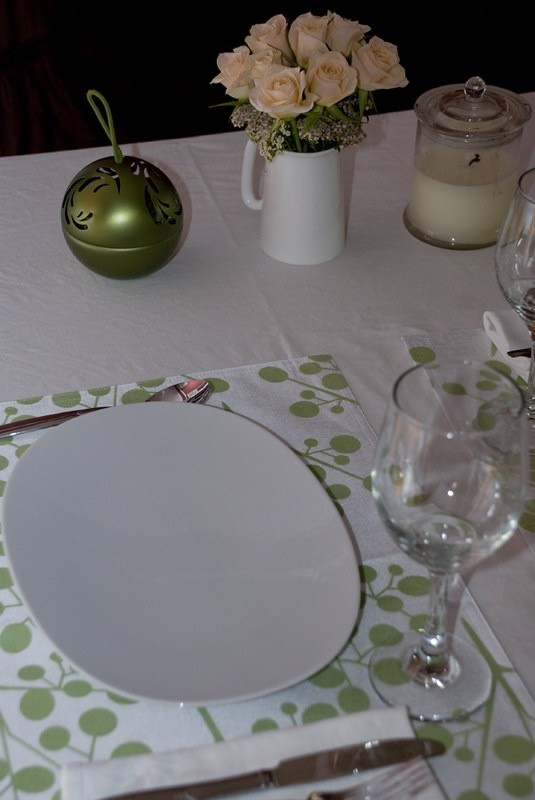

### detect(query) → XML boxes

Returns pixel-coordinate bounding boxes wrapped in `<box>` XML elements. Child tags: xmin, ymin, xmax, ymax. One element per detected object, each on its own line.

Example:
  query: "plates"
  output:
<box><xmin>1</xmin><ymin>402</ymin><xmax>361</xmax><ymax>707</ymax></box>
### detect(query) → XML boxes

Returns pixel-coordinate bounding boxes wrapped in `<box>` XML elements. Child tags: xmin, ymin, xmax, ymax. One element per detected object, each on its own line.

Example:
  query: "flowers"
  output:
<box><xmin>209</xmin><ymin>9</ymin><xmax>411</xmax><ymax>163</ymax></box>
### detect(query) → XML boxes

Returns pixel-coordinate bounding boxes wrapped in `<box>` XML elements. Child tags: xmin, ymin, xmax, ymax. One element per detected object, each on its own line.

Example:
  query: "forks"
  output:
<box><xmin>305</xmin><ymin>754</ymin><xmax>434</xmax><ymax>799</ymax></box>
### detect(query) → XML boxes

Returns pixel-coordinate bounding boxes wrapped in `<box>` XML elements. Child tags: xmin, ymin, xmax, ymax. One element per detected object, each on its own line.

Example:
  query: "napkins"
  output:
<box><xmin>56</xmin><ymin>703</ymin><xmax>443</xmax><ymax>800</ymax></box>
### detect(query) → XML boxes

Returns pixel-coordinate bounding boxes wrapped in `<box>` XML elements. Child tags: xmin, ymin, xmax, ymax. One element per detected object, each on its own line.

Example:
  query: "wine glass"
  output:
<box><xmin>367</xmin><ymin>358</ymin><xmax>529</xmax><ymax>724</ymax></box>
<box><xmin>477</xmin><ymin>166</ymin><xmax>535</xmax><ymax>459</ymax></box>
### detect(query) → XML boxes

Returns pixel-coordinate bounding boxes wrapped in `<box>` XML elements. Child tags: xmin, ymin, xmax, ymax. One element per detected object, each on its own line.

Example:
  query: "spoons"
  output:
<box><xmin>0</xmin><ymin>379</ymin><xmax>215</xmax><ymax>440</ymax></box>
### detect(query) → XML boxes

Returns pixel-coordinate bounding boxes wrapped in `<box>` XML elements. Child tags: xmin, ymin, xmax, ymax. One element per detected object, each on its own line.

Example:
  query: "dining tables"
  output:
<box><xmin>0</xmin><ymin>91</ymin><xmax>535</xmax><ymax>800</ymax></box>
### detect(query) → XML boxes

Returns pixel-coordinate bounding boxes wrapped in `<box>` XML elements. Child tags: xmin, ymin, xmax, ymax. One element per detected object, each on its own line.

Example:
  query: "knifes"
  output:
<box><xmin>103</xmin><ymin>737</ymin><xmax>448</xmax><ymax>800</ymax></box>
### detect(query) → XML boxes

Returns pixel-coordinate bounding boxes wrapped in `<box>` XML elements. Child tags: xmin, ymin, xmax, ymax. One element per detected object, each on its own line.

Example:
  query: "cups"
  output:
<box><xmin>402</xmin><ymin>76</ymin><xmax>532</xmax><ymax>251</ymax></box>
